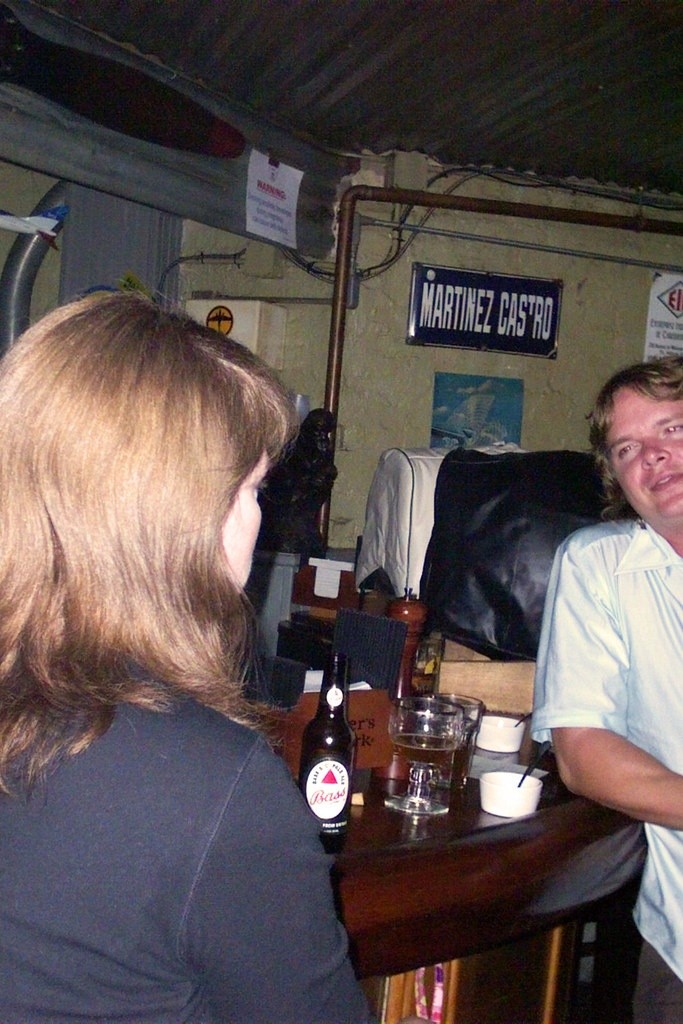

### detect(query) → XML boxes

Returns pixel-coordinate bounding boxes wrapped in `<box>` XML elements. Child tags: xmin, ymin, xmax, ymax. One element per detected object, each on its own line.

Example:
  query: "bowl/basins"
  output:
<box><xmin>477</xmin><ymin>771</ymin><xmax>543</xmax><ymax>818</ymax></box>
<box><xmin>476</xmin><ymin>716</ymin><xmax>526</xmax><ymax>753</ymax></box>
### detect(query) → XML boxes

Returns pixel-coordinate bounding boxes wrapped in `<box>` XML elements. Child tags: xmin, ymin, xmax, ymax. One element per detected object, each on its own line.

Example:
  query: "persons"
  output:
<box><xmin>530</xmin><ymin>353</ymin><xmax>683</xmax><ymax>1024</ymax></box>
<box><xmin>255</xmin><ymin>408</ymin><xmax>338</xmax><ymax>565</ymax></box>
<box><xmin>0</xmin><ymin>288</ymin><xmax>372</xmax><ymax>1024</ymax></box>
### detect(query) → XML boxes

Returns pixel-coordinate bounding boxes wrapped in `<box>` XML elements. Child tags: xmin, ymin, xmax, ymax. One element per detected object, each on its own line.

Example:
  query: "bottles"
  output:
<box><xmin>297</xmin><ymin>651</ymin><xmax>357</xmax><ymax>855</ymax></box>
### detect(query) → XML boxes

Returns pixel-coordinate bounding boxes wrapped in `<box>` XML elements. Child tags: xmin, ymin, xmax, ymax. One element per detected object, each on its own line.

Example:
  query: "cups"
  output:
<box><xmin>410</xmin><ymin>639</ymin><xmax>444</xmax><ymax>696</ymax></box>
<box><xmin>420</xmin><ymin>692</ymin><xmax>483</xmax><ymax>790</ymax></box>
<box><xmin>383</xmin><ymin>697</ymin><xmax>464</xmax><ymax>815</ymax></box>
